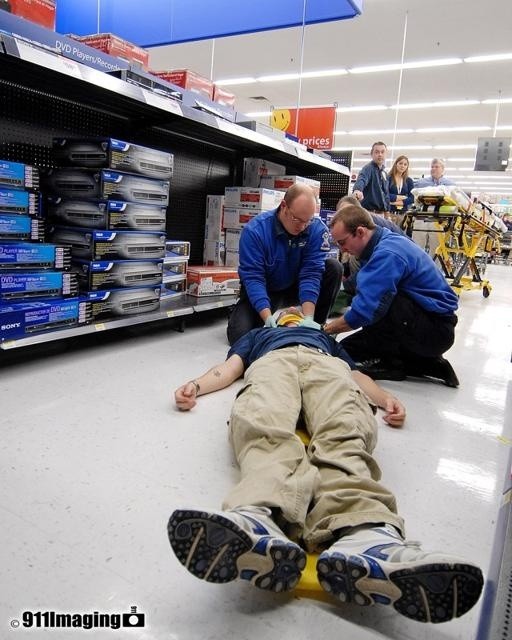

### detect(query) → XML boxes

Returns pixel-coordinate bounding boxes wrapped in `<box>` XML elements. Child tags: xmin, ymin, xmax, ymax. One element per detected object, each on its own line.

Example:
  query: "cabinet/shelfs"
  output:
<box><xmin>0</xmin><ymin>31</ymin><xmax>354</xmax><ymax>352</ymax></box>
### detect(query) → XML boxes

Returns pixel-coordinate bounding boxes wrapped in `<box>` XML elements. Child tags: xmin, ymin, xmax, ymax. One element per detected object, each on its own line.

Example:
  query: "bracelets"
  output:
<box><xmin>188</xmin><ymin>381</ymin><xmax>199</xmax><ymax>398</ymax></box>
<box><xmin>320</xmin><ymin>323</ymin><xmax>327</xmax><ymax>332</ymax></box>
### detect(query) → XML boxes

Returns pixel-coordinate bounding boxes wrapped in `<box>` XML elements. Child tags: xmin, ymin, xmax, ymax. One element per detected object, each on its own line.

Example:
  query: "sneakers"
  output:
<box><xmin>167</xmin><ymin>504</ymin><xmax>306</xmax><ymax>593</ymax></box>
<box><xmin>355</xmin><ymin>359</ymin><xmax>406</xmax><ymax>381</ymax></box>
<box><xmin>316</xmin><ymin>524</ymin><xmax>484</xmax><ymax>624</ymax></box>
<box><xmin>424</xmin><ymin>355</ymin><xmax>459</xmax><ymax>387</ymax></box>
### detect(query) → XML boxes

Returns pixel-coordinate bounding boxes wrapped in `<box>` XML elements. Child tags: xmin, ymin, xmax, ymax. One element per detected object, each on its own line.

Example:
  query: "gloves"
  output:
<box><xmin>265</xmin><ymin>315</ymin><xmax>277</xmax><ymax>328</ymax></box>
<box><xmin>299</xmin><ymin>316</ymin><xmax>321</xmax><ymax>331</ymax></box>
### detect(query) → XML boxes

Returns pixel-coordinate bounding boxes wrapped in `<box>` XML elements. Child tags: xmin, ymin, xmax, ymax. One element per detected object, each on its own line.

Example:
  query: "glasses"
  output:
<box><xmin>336</xmin><ymin>226</ymin><xmax>366</xmax><ymax>246</ymax></box>
<box><xmin>286</xmin><ymin>206</ymin><xmax>313</xmax><ymax>223</ymax></box>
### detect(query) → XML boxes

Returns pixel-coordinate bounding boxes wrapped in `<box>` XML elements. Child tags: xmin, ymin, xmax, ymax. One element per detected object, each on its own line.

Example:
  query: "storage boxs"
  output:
<box><xmin>1</xmin><ymin>9</ymin><xmax>334</xmax><ymax>344</ymax></box>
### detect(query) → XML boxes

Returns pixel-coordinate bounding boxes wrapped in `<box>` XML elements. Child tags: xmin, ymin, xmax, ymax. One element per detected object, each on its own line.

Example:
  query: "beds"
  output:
<box><xmin>399</xmin><ymin>185</ymin><xmax>508</xmax><ymax>298</ymax></box>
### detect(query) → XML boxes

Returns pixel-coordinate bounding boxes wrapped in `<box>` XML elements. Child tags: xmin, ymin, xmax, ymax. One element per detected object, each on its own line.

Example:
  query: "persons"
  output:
<box><xmin>337</xmin><ymin>196</ymin><xmax>415</xmax><ymax>304</ymax></box>
<box><xmin>351</xmin><ymin>141</ymin><xmax>390</xmax><ymax>224</ymax></box>
<box><xmin>384</xmin><ymin>155</ymin><xmax>414</xmax><ymax>238</ymax></box>
<box><xmin>299</xmin><ymin>206</ymin><xmax>459</xmax><ymax>387</ymax></box>
<box><xmin>175</xmin><ymin>306</ymin><xmax>484</xmax><ymax>624</ymax></box>
<box><xmin>411</xmin><ymin>159</ymin><xmax>459</xmax><ymax>278</ymax></box>
<box><xmin>227</xmin><ymin>183</ymin><xmax>344</xmax><ymax>348</ymax></box>
<box><xmin>501</xmin><ymin>213</ymin><xmax>511</xmax><ymax>264</ymax></box>
